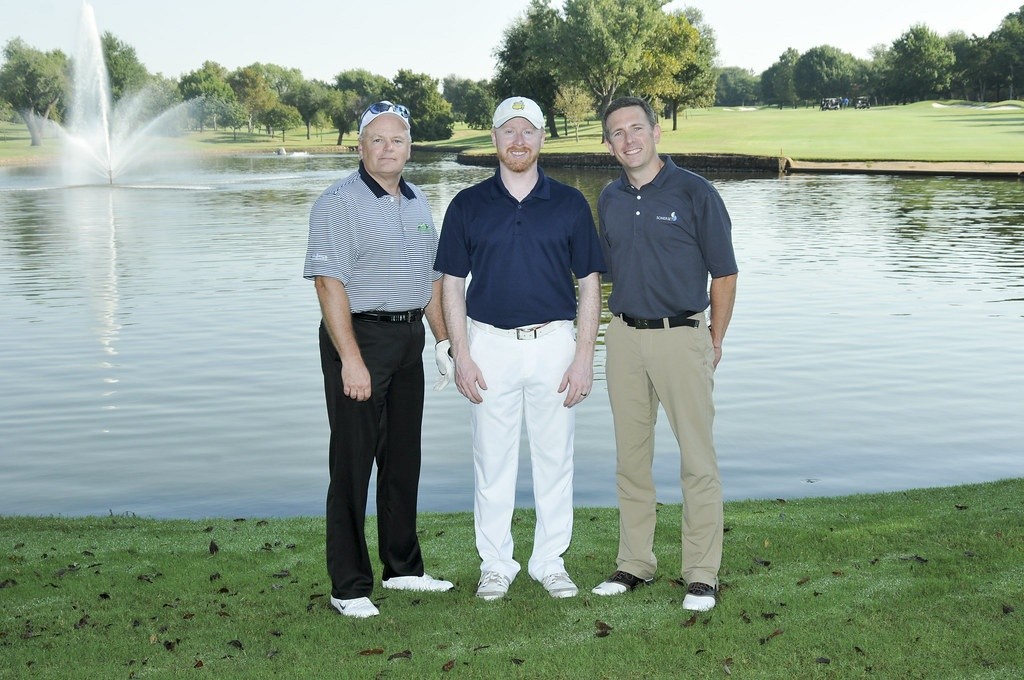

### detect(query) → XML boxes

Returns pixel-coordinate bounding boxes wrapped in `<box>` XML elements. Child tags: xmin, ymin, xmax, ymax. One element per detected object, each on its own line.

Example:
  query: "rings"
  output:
<box><xmin>581</xmin><ymin>393</ymin><xmax>586</xmax><ymax>396</ymax></box>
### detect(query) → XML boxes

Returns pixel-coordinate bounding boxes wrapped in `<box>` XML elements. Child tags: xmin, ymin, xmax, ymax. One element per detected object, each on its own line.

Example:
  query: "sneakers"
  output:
<box><xmin>331</xmin><ymin>594</ymin><xmax>379</xmax><ymax>618</ymax></box>
<box><xmin>540</xmin><ymin>571</ymin><xmax>578</xmax><ymax>597</ymax></box>
<box><xmin>683</xmin><ymin>582</ymin><xmax>716</xmax><ymax>611</ymax></box>
<box><xmin>591</xmin><ymin>569</ymin><xmax>653</xmax><ymax>596</ymax></box>
<box><xmin>382</xmin><ymin>572</ymin><xmax>454</xmax><ymax>593</ymax></box>
<box><xmin>476</xmin><ymin>570</ymin><xmax>511</xmax><ymax>598</ymax></box>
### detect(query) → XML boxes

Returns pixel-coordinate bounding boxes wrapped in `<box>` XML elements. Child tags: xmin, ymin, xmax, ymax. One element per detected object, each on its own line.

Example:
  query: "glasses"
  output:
<box><xmin>358</xmin><ymin>103</ymin><xmax>410</xmax><ymax>127</ymax></box>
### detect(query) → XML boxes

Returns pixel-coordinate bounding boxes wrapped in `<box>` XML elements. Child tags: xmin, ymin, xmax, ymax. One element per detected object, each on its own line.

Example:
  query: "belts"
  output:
<box><xmin>472</xmin><ymin>319</ymin><xmax>568</xmax><ymax>340</ymax></box>
<box><xmin>618</xmin><ymin>310</ymin><xmax>700</xmax><ymax>329</ymax></box>
<box><xmin>351</xmin><ymin>310</ymin><xmax>425</xmax><ymax>323</ymax></box>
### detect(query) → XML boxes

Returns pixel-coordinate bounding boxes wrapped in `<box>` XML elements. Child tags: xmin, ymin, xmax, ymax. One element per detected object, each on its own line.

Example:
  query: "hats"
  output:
<box><xmin>493</xmin><ymin>96</ymin><xmax>545</xmax><ymax>129</ymax></box>
<box><xmin>359</xmin><ymin>100</ymin><xmax>410</xmax><ymax>137</ymax></box>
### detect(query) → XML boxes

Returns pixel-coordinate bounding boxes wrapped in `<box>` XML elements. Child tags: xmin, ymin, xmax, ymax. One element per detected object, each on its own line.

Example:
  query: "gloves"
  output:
<box><xmin>434</xmin><ymin>337</ymin><xmax>455</xmax><ymax>390</ymax></box>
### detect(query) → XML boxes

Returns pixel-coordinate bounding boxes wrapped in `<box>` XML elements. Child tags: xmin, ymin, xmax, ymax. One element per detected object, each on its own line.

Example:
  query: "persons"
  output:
<box><xmin>431</xmin><ymin>97</ymin><xmax>608</xmax><ymax>602</ymax></box>
<box><xmin>590</xmin><ymin>97</ymin><xmax>739</xmax><ymax>612</ymax></box>
<box><xmin>302</xmin><ymin>100</ymin><xmax>457</xmax><ymax>619</ymax></box>
<box><xmin>819</xmin><ymin>97</ymin><xmax>849</xmax><ymax>111</ymax></box>
